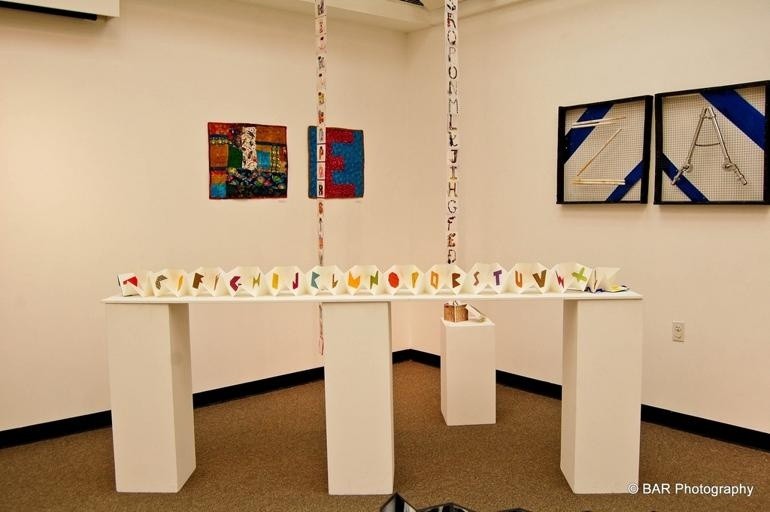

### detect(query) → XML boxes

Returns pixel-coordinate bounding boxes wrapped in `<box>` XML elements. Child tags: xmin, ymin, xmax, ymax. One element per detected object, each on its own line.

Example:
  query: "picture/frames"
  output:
<box><xmin>556</xmin><ymin>80</ymin><xmax>769</xmax><ymax>205</ymax></box>
